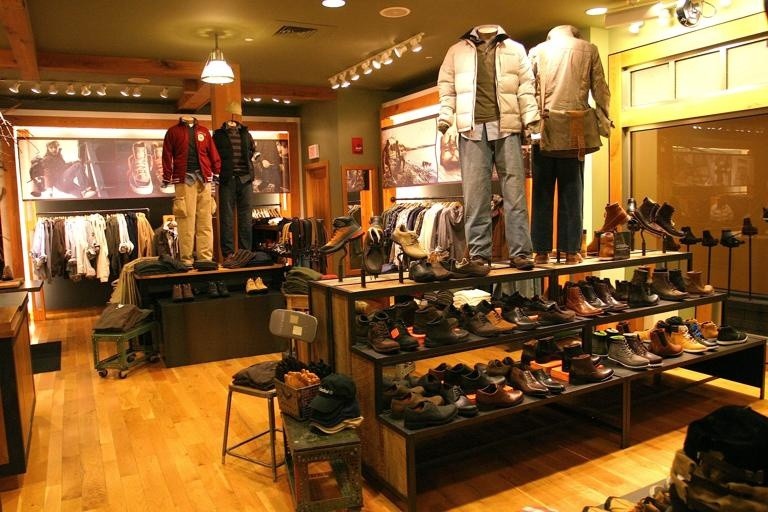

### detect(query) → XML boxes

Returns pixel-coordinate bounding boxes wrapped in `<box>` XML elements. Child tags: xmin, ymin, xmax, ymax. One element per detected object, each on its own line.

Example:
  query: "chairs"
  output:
<box><xmin>221</xmin><ymin>309</ymin><xmax>319</xmax><ymax>482</ymax></box>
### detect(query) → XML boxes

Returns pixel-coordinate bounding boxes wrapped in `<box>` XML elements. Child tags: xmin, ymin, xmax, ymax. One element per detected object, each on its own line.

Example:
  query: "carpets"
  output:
<box><xmin>30</xmin><ymin>341</ymin><xmax>61</xmax><ymax>374</ymax></box>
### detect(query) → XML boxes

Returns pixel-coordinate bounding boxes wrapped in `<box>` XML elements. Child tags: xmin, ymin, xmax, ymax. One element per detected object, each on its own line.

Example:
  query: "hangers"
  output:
<box><xmin>390</xmin><ymin>201</ymin><xmax>463</xmax><ymax>208</ymax></box>
<box><xmin>250</xmin><ymin>207</ymin><xmax>283</xmax><ymax>218</ymax></box>
<box><xmin>37</xmin><ymin>216</ymin><xmax>69</xmax><ymax>220</ymax></box>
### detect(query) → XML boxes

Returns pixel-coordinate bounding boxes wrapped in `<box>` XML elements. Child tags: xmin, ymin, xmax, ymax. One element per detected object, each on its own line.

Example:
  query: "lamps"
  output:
<box><xmin>327</xmin><ymin>32</ymin><xmax>425</xmax><ymax>90</ymax></box>
<box><xmin>8</xmin><ymin>80</ymin><xmax>169</xmax><ymax>99</ymax></box>
<box><xmin>675</xmin><ymin>0</ymin><xmax>705</xmax><ymax>27</ymax></box>
<box><xmin>200</xmin><ymin>33</ymin><xmax>236</xmax><ymax>85</ymax></box>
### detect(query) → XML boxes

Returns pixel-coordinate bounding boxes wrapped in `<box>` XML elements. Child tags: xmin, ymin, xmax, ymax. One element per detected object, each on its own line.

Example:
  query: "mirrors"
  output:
<box><xmin>341</xmin><ymin>165</ymin><xmax>380</xmax><ymax>277</ymax></box>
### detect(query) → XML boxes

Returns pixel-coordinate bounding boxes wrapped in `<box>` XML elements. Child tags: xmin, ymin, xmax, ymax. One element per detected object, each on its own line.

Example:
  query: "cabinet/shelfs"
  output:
<box><xmin>308</xmin><ymin>250</ymin><xmax>767</xmax><ymax>512</ymax></box>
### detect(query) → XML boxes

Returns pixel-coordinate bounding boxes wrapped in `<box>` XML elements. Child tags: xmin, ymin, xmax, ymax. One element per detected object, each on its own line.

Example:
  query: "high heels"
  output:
<box><xmin>588</xmin><ymin>200</ymin><xmax>632</xmax><ymax>232</ymax></box>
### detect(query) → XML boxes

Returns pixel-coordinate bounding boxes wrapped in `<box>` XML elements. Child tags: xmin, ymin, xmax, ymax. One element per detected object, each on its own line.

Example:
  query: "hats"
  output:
<box><xmin>305</xmin><ymin>372</ymin><xmax>365</xmax><ymax>436</ymax></box>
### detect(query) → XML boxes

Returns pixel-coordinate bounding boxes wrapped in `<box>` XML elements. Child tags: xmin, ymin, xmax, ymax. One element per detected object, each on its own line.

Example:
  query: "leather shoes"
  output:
<box><xmin>206</xmin><ymin>279</ymin><xmax>230</xmax><ymax>299</ymax></box>
<box><xmin>171</xmin><ymin>282</ymin><xmax>196</xmax><ymax>303</ymax></box>
<box><xmin>507</xmin><ymin>253</ymin><xmax>550</xmax><ymax>271</ymax></box>
<box><xmin>381</xmin><ymin>355</ymin><xmax>567</xmax><ymax>431</ymax></box>
<box><xmin>563</xmin><ymin>251</ymin><xmax>584</xmax><ymax>266</ymax></box>
<box><xmin>244</xmin><ymin>276</ymin><xmax>268</xmax><ymax>295</ymax></box>
<box><xmin>361</xmin><ymin>224</ymin><xmax>385</xmax><ymax>275</ymax></box>
<box><xmin>390</xmin><ymin>223</ymin><xmax>429</xmax><ymax>261</ymax></box>
<box><xmin>445</xmin><ymin>288</ymin><xmax>577</xmax><ymax>349</ymax></box>
<box><xmin>407</xmin><ymin>253</ymin><xmax>491</xmax><ymax>283</ymax></box>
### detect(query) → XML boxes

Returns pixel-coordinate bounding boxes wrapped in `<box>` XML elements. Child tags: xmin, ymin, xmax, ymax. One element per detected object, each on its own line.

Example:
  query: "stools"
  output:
<box><xmin>283</xmin><ymin>410</ymin><xmax>364</xmax><ymax>512</ymax></box>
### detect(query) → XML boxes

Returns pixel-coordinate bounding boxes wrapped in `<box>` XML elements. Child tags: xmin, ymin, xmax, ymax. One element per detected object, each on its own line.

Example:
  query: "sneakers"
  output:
<box><xmin>315</xmin><ymin>215</ymin><xmax>366</xmax><ymax>254</ymax></box>
<box><xmin>125</xmin><ymin>140</ymin><xmax>176</xmax><ymax>195</ymax></box>
<box><xmin>579</xmin><ymin>475</ymin><xmax>686</xmax><ymax>512</ymax></box>
<box><xmin>585</xmin><ymin>229</ymin><xmax>631</xmax><ymax>261</ymax></box>
<box><xmin>80</xmin><ymin>187</ymin><xmax>97</xmax><ymax>199</ymax></box>
<box><xmin>1</xmin><ymin>265</ymin><xmax>15</xmax><ymax>281</ymax></box>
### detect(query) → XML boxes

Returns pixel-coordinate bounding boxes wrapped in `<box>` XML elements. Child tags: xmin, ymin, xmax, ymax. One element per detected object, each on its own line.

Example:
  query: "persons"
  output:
<box><xmin>213</xmin><ymin>119</ymin><xmax>258</xmax><ymax>264</ymax></box>
<box><xmin>435</xmin><ymin>24</ymin><xmax>536</xmax><ymax>271</ymax></box>
<box><xmin>160</xmin><ymin>114</ymin><xmax>221</xmax><ymax>272</ymax></box>
<box><xmin>527</xmin><ymin>24</ymin><xmax>610</xmax><ymax>267</ymax></box>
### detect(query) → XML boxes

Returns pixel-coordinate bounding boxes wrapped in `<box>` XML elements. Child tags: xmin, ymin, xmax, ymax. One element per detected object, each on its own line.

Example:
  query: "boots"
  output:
<box><xmin>590</xmin><ymin>316</ymin><xmax>750</xmax><ymax>371</ymax></box>
<box><xmin>560</xmin><ymin>341</ymin><xmax>614</xmax><ymax>386</ymax></box>
<box><xmin>632</xmin><ymin>195</ymin><xmax>685</xmax><ymax>239</ymax></box>
<box><xmin>386</xmin><ymin>300</ymin><xmax>470</xmax><ymax>349</ymax></box>
<box><xmin>557</xmin><ymin>265</ymin><xmax>715</xmax><ymax>318</ymax></box>
<box><xmin>354</xmin><ymin>311</ymin><xmax>420</xmax><ymax>353</ymax></box>
<box><xmin>660</xmin><ymin>207</ymin><xmax>767</xmax><ymax>253</ymax></box>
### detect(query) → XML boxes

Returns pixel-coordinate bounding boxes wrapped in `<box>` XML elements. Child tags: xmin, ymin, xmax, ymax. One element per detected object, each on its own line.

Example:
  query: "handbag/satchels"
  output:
<box><xmin>536</xmin><ymin>105</ymin><xmax>603</xmax><ymax>161</ymax></box>
